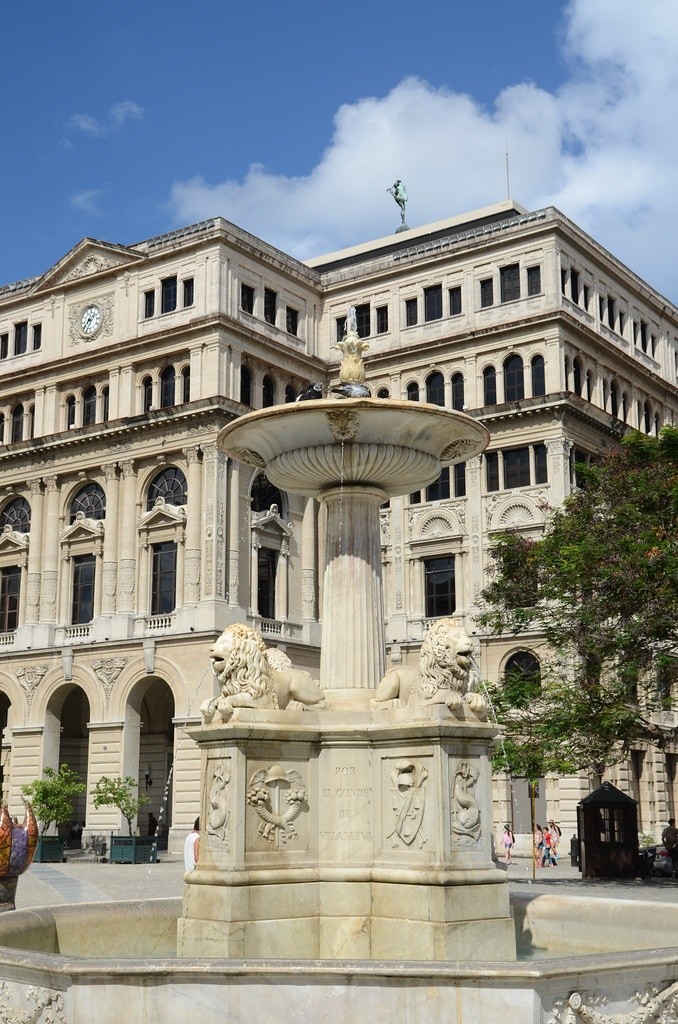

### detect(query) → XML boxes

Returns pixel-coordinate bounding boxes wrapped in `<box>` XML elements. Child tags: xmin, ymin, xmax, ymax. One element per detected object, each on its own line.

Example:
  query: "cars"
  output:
<box><xmin>652</xmin><ymin>846</ymin><xmax>673</xmax><ymax>874</ymax></box>
<box><xmin>639</xmin><ymin>843</ymin><xmax>664</xmax><ymax>868</ymax></box>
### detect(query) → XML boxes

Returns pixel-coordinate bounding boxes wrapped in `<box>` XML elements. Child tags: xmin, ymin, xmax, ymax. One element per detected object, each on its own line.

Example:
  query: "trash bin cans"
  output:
<box><xmin>97</xmin><ymin>842</ymin><xmax>106</xmax><ymax>856</ymax></box>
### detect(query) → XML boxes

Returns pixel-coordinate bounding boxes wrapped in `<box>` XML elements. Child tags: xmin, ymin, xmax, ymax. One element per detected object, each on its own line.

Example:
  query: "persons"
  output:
<box><xmin>147</xmin><ymin>812</ymin><xmax>157</xmax><ymax>837</ymax></box>
<box><xmin>63</xmin><ymin>821</ymin><xmax>85</xmax><ymax>847</ymax></box>
<box><xmin>500</xmin><ymin>824</ymin><xmax>514</xmax><ymax>865</ymax></box>
<box><xmin>184</xmin><ymin>816</ymin><xmax>200</xmax><ymax>872</ymax></box>
<box><xmin>662</xmin><ymin>819</ymin><xmax>678</xmax><ymax>879</ymax></box>
<box><xmin>534</xmin><ymin>819</ymin><xmax>560</xmax><ymax>867</ymax></box>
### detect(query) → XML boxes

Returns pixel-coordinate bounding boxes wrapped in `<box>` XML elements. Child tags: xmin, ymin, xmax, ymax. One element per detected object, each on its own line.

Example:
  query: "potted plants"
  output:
<box><xmin>93</xmin><ymin>775</ymin><xmax>154</xmax><ymax>865</ymax></box>
<box><xmin>23</xmin><ymin>764</ymin><xmax>85</xmax><ymax>862</ymax></box>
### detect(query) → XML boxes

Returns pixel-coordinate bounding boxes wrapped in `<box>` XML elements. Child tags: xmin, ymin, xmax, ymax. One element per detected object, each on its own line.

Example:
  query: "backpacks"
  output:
<box><xmin>554</xmin><ymin>825</ymin><xmax>561</xmax><ymax>836</ymax></box>
<box><xmin>508</xmin><ymin>830</ymin><xmax>515</xmax><ymax>843</ymax></box>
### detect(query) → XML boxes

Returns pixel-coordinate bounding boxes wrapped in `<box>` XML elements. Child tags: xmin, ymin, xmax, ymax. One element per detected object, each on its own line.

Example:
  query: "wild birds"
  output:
<box><xmin>295</xmin><ymin>383</ymin><xmax>324</xmax><ymax>402</ymax></box>
<box><xmin>331</xmin><ymin>384</ymin><xmax>372</xmax><ymax>398</ymax></box>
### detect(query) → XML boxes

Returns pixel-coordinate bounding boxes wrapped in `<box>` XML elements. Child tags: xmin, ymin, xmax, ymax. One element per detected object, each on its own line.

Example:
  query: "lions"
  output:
<box><xmin>373</xmin><ymin>617</ymin><xmax>488</xmax><ymax>713</ymax></box>
<box><xmin>199</xmin><ymin>623</ymin><xmax>327</xmax><ymax>725</ymax></box>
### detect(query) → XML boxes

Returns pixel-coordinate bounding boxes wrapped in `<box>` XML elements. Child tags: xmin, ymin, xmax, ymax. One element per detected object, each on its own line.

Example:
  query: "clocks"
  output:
<box><xmin>78</xmin><ymin>302</ymin><xmax>102</xmax><ymax>338</ymax></box>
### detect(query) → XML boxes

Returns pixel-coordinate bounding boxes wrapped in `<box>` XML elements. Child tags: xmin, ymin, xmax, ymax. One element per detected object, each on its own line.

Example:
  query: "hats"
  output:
<box><xmin>504</xmin><ymin>824</ymin><xmax>510</xmax><ymax>829</ymax></box>
<box><xmin>547</xmin><ymin>819</ymin><xmax>554</xmax><ymax>823</ymax></box>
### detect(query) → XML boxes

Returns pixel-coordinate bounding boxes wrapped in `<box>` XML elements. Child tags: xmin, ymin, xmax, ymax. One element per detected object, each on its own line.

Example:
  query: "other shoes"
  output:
<box><xmin>548</xmin><ymin>864</ymin><xmax>553</xmax><ymax>866</ymax></box>
<box><xmin>540</xmin><ymin>864</ymin><xmax>544</xmax><ymax>866</ymax></box>
